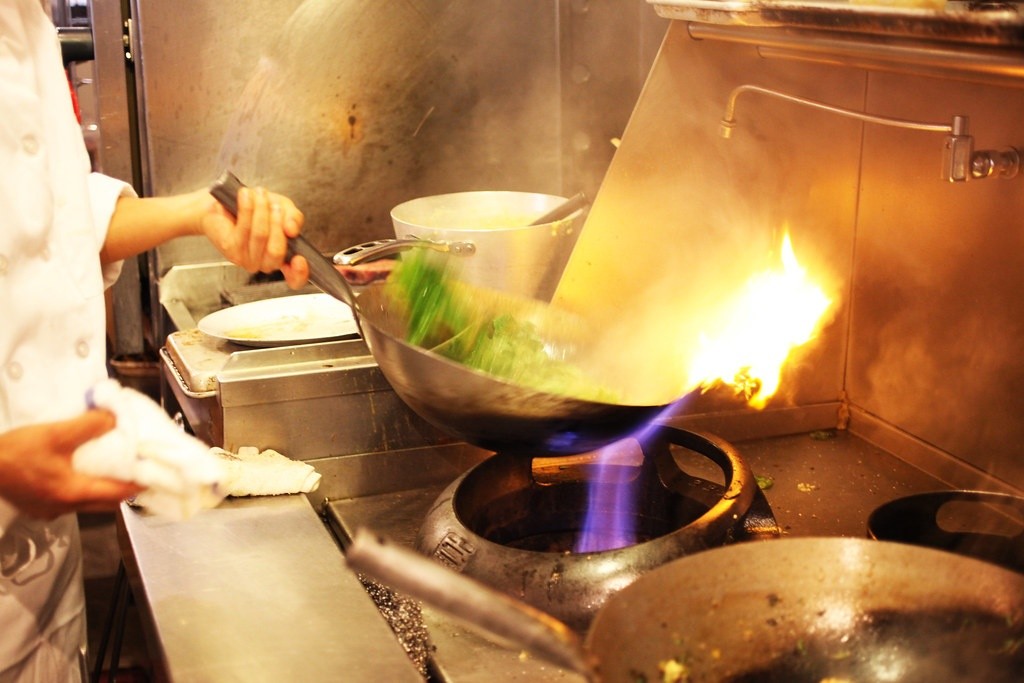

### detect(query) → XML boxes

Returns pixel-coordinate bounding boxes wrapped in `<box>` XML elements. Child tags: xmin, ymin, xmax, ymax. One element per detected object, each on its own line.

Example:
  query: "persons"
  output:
<box><xmin>0</xmin><ymin>1</ymin><xmax>309</xmax><ymax>683</ymax></box>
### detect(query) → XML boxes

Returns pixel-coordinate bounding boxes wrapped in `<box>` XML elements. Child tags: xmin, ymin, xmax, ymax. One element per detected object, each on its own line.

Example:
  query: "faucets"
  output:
<box><xmin>718</xmin><ymin>84</ymin><xmax>967</xmax><ymax>139</ymax></box>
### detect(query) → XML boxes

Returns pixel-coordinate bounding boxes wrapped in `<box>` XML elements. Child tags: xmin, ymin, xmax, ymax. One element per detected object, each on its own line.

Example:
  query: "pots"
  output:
<box><xmin>346</xmin><ymin>524</ymin><xmax>1024</xmax><ymax>683</ymax></box>
<box><xmin>210</xmin><ymin>171</ymin><xmax>687</xmax><ymax>457</ymax></box>
<box><xmin>334</xmin><ymin>192</ymin><xmax>582</xmax><ymax>302</ymax></box>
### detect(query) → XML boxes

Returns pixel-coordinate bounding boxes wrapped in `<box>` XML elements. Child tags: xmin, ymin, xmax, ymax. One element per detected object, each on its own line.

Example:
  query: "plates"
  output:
<box><xmin>199</xmin><ymin>293</ymin><xmax>358</xmax><ymax>348</ymax></box>
